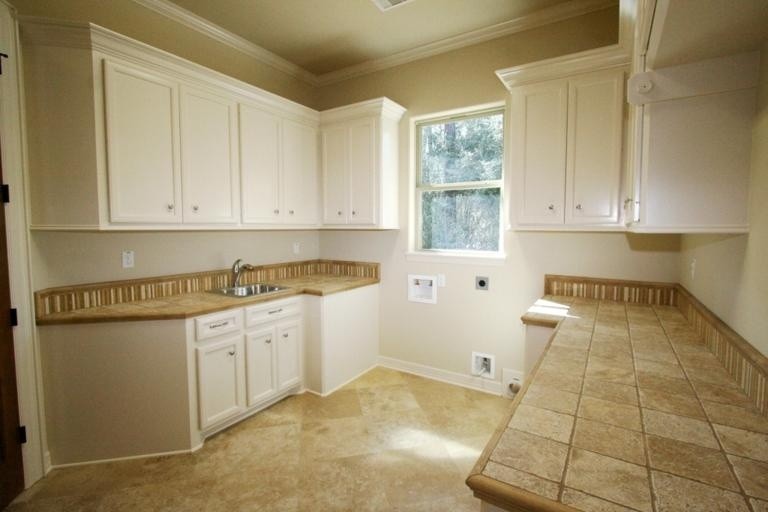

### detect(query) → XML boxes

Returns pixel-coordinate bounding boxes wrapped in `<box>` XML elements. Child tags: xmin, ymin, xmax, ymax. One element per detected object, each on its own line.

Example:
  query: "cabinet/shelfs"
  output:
<box><xmin>237</xmin><ymin>98</ymin><xmax>319</xmax><ymax>230</ymax></box>
<box><xmin>38</xmin><ymin>307</ymin><xmax>246</xmax><ymax>468</ymax></box>
<box><xmin>321</xmin><ymin>116</ymin><xmax>402</xmax><ymax>231</ymax></box>
<box><xmin>24</xmin><ymin>37</ymin><xmax>236</xmax><ymax>230</ymax></box>
<box><xmin>245</xmin><ymin>294</ymin><xmax>310</xmax><ymax>409</ymax></box>
<box><xmin>506</xmin><ymin>0</ymin><xmax>749</xmax><ymax>231</ymax></box>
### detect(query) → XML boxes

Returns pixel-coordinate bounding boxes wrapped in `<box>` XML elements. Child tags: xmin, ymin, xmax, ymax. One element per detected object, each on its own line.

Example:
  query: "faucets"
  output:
<box><xmin>232</xmin><ymin>258</ymin><xmax>253</xmax><ymax>288</ymax></box>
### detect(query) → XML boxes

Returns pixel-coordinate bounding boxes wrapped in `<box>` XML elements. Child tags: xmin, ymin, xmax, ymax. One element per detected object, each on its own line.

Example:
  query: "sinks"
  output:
<box><xmin>204</xmin><ymin>284</ymin><xmax>291</xmax><ymax>297</ymax></box>
